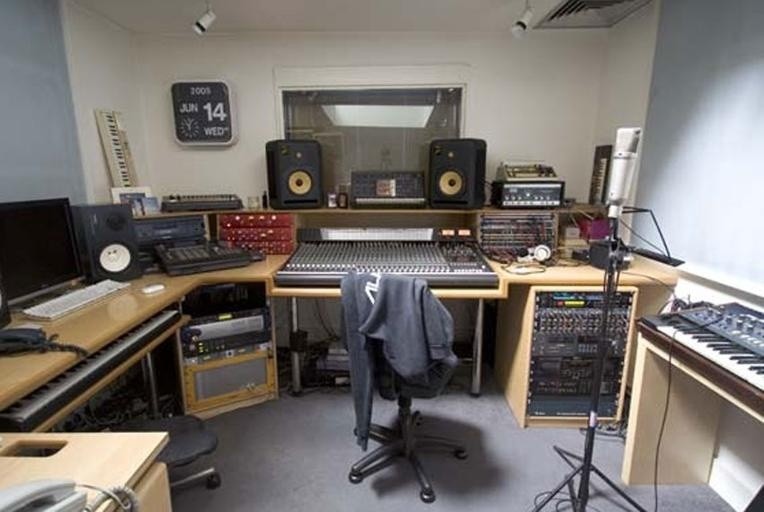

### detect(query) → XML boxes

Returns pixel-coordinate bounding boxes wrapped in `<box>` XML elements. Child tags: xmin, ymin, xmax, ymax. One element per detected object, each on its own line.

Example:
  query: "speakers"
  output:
<box><xmin>428</xmin><ymin>138</ymin><xmax>487</xmax><ymax>210</ymax></box>
<box><xmin>266</xmin><ymin>140</ymin><xmax>323</xmax><ymax>209</ymax></box>
<box><xmin>71</xmin><ymin>203</ymin><xmax>142</xmax><ymax>285</ymax></box>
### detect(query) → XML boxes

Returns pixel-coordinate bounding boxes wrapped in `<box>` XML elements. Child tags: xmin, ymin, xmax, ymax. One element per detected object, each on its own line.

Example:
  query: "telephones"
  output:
<box><xmin>0</xmin><ymin>474</ymin><xmax>88</xmax><ymax>511</ymax></box>
<box><xmin>0</xmin><ymin>328</ymin><xmax>46</xmax><ymax>355</ymax></box>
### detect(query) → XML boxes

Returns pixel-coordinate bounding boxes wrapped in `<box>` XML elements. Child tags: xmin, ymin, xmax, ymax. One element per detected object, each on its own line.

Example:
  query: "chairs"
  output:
<box><xmin>336</xmin><ymin>274</ymin><xmax>472</xmax><ymax>504</ymax></box>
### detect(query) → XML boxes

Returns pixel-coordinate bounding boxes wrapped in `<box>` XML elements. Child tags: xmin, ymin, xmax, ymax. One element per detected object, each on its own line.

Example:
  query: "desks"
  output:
<box><xmin>1</xmin><ymin>253</ymin><xmax>677</xmax><ymax>434</ymax></box>
<box><xmin>622</xmin><ymin>327</ymin><xmax>764</xmax><ymax>489</ymax></box>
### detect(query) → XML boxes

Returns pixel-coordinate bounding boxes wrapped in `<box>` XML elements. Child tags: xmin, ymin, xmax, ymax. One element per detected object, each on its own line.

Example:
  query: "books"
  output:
<box><xmin>479</xmin><ymin>211</ymin><xmax>555</xmax><ymax>254</ymax></box>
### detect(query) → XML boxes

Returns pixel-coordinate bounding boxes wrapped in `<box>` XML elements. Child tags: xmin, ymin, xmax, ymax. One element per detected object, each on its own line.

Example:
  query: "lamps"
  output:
<box><xmin>512</xmin><ymin>0</ymin><xmax>535</xmax><ymax>33</ymax></box>
<box><xmin>192</xmin><ymin>2</ymin><xmax>216</xmax><ymax>34</ymax></box>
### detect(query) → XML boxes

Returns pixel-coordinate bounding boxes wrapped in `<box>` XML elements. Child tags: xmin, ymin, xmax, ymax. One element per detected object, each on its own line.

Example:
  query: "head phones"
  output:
<box><xmin>517</xmin><ymin>244</ymin><xmax>551</xmax><ymax>263</ymax></box>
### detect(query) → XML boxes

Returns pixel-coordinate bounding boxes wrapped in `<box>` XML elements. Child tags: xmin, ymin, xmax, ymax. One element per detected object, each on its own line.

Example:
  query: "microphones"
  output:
<box><xmin>607</xmin><ymin>127</ymin><xmax>644</xmax><ymax>219</ymax></box>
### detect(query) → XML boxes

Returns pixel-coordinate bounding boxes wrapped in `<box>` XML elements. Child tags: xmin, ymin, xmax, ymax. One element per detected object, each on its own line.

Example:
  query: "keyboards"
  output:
<box><xmin>21</xmin><ymin>278</ymin><xmax>132</xmax><ymax>320</ymax></box>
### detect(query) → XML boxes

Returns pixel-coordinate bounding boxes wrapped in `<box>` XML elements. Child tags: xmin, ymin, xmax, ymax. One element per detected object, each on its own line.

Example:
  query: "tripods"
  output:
<box><xmin>532</xmin><ymin>218</ymin><xmax>685</xmax><ymax>512</ymax></box>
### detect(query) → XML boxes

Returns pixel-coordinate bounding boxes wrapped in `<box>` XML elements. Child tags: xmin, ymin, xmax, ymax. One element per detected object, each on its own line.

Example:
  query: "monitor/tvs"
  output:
<box><xmin>0</xmin><ymin>197</ymin><xmax>86</xmax><ymax>306</ymax></box>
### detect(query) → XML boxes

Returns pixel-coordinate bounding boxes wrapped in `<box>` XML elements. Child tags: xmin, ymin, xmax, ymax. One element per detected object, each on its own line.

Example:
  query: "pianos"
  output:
<box><xmin>640</xmin><ymin>302</ymin><xmax>764</xmax><ymax>398</ymax></box>
<box><xmin>0</xmin><ymin>308</ymin><xmax>181</xmax><ymax>433</ymax></box>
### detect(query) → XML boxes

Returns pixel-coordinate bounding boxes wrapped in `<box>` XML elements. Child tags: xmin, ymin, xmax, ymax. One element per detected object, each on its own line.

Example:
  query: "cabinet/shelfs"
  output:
<box><xmin>0</xmin><ymin>433</ymin><xmax>175</xmax><ymax>512</ymax></box>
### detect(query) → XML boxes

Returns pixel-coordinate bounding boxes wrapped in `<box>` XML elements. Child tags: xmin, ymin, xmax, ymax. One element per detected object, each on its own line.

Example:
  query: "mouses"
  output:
<box><xmin>142</xmin><ymin>284</ymin><xmax>165</xmax><ymax>294</ymax></box>
